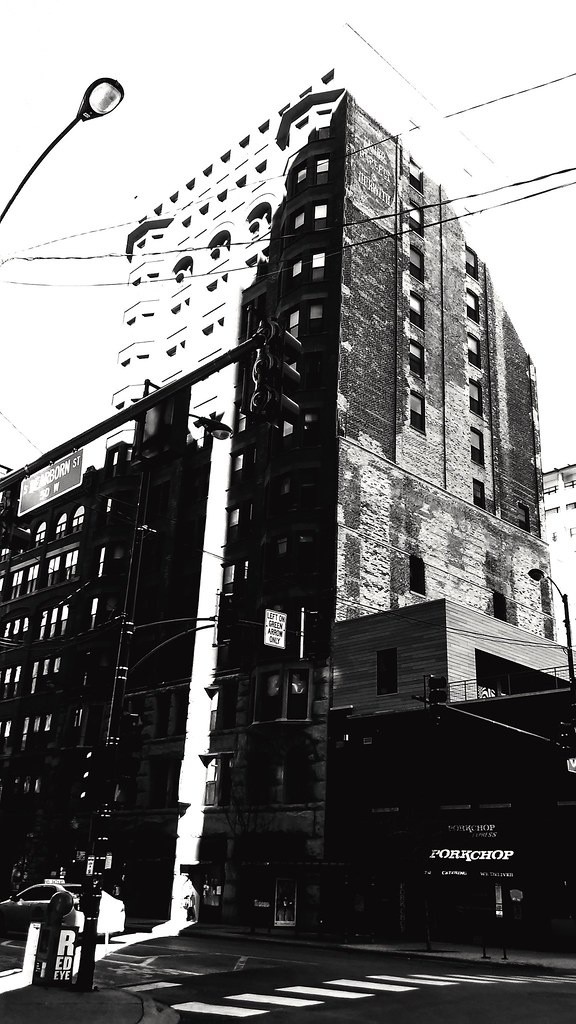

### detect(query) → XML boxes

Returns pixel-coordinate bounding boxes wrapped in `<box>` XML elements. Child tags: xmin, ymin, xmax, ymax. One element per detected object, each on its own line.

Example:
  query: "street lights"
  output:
<box><xmin>527</xmin><ymin>568</ymin><xmax>576</xmax><ymax>720</ymax></box>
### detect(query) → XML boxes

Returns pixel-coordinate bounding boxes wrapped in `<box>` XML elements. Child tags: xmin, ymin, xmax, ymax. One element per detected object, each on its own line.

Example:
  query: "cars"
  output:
<box><xmin>0</xmin><ymin>879</ymin><xmax>126</xmax><ymax>944</ymax></box>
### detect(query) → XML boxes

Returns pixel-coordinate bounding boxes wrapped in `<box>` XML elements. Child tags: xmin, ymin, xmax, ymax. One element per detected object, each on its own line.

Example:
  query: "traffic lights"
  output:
<box><xmin>427</xmin><ymin>673</ymin><xmax>448</xmax><ymax>728</ymax></box>
<box><xmin>248</xmin><ymin>318</ymin><xmax>302</xmax><ymax>437</ymax></box>
<box><xmin>303</xmin><ymin>608</ymin><xmax>320</xmax><ymax>659</ymax></box>
<box><xmin>217</xmin><ymin>591</ymin><xmax>237</xmax><ymax>645</ymax></box>
